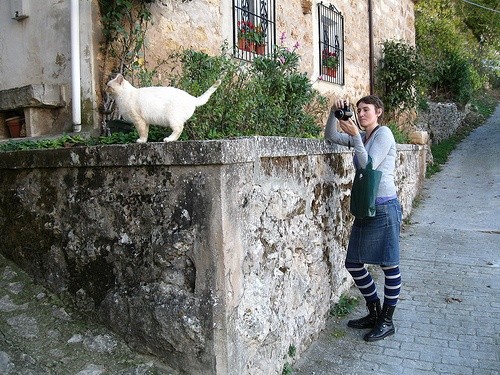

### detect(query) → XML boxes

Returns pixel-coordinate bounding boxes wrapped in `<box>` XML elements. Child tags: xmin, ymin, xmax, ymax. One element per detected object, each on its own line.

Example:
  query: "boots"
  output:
<box><xmin>363</xmin><ymin>302</ymin><xmax>398</xmax><ymax>344</ymax></box>
<box><xmin>347</xmin><ymin>299</ymin><xmax>381</xmax><ymax>329</ymax></box>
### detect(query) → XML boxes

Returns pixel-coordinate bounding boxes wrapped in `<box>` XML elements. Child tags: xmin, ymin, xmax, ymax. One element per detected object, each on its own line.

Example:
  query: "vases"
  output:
<box><xmin>256</xmin><ymin>44</ymin><xmax>266</xmax><ymax>56</ymax></box>
<box><xmin>238</xmin><ymin>38</ymin><xmax>248</xmax><ymax>50</ymax></box>
<box><xmin>246</xmin><ymin>42</ymin><xmax>256</xmax><ymax>52</ymax></box>
<box><xmin>323</xmin><ymin>66</ymin><xmax>336</xmax><ymax>77</ymax></box>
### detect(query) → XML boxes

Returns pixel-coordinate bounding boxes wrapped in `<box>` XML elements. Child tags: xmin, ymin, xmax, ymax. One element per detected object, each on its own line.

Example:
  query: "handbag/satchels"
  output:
<box><xmin>350</xmin><ymin>154</ymin><xmax>382</xmax><ymax>220</ymax></box>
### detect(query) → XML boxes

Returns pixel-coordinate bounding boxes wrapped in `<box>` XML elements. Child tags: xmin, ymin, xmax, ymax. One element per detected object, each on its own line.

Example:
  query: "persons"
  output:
<box><xmin>324</xmin><ymin>96</ymin><xmax>401</xmax><ymax>342</ymax></box>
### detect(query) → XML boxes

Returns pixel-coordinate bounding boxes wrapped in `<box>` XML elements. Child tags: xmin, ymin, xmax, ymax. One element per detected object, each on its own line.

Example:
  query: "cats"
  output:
<box><xmin>108</xmin><ymin>68</ymin><xmax>228</xmax><ymax>143</ymax></box>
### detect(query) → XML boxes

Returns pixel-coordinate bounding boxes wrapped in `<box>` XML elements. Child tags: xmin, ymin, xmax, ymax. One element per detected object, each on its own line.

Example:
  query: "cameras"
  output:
<box><xmin>334</xmin><ymin>103</ymin><xmax>352</xmax><ymax>121</ymax></box>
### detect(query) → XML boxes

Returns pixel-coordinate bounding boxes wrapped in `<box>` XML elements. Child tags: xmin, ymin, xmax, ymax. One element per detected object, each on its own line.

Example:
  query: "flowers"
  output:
<box><xmin>322</xmin><ymin>48</ymin><xmax>338</xmax><ymax>68</ymax></box>
<box><xmin>238</xmin><ymin>20</ymin><xmax>267</xmax><ymax>45</ymax></box>
